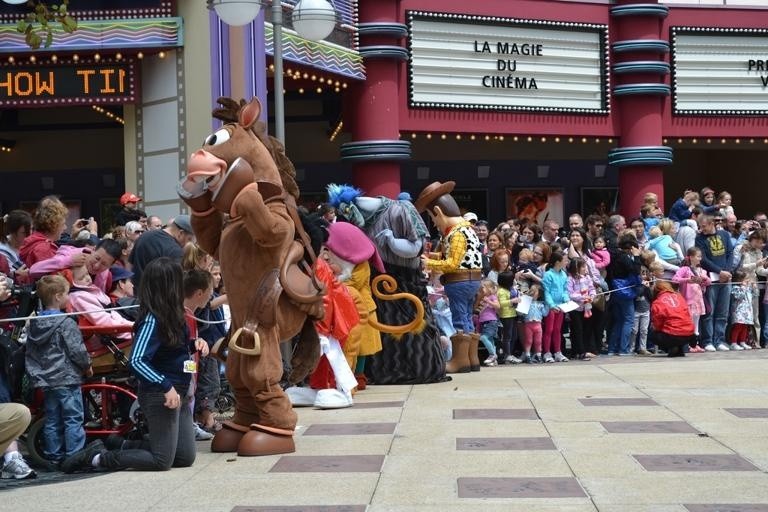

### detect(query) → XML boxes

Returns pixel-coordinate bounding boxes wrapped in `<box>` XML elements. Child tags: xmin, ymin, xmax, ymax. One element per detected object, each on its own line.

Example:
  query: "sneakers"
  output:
<box><xmin>104</xmin><ymin>435</ymin><xmax>124</xmax><ymax>450</ymax></box>
<box><xmin>486</xmin><ymin>359</ymin><xmax>499</xmax><ymax>367</ymax></box>
<box><xmin>505</xmin><ymin>354</ymin><xmax>524</xmax><ymax>365</ymax></box>
<box><xmin>483</xmin><ymin>353</ymin><xmax>498</xmax><ymax>364</ymax></box>
<box><xmin>571</xmin><ymin>349</ymin><xmax>654</xmax><ymax>361</ymax></box>
<box><xmin>688</xmin><ymin>341</ymin><xmax>763</xmax><ymax>353</ymax></box>
<box><xmin>543</xmin><ymin>352</ymin><xmax>556</xmax><ymax>363</ymax></box>
<box><xmin>1</xmin><ymin>453</ymin><xmax>39</xmax><ymax>480</ymax></box>
<box><xmin>60</xmin><ymin>438</ymin><xmax>105</xmax><ymax>472</ymax></box>
<box><xmin>520</xmin><ymin>352</ymin><xmax>544</xmax><ymax>364</ymax></box>
<box><xmin>554</xmin><ymin>351</ymin><xmax>570</xmax><ymax>362</ymax></box>
<box><xmin>193</xmin><ymin>423</ymin><xmax>214</xmax><ymax>441</ymax></box>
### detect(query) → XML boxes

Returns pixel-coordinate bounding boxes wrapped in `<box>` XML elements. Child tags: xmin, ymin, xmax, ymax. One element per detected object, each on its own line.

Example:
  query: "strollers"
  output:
<box><xmin>9</xmin><ymin>308</ymin><xmax>240</xmax><ymax>470</ymax></box>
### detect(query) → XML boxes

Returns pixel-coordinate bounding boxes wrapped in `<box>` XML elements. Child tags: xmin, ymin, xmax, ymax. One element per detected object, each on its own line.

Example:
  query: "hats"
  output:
<box><xmin>174</xmin><ymin>215</ymin><xmax>193</xmax><ymax>234</ymax></box>
<box><xmin>119</xmin><ymin>191</ymin><xmax>144</xmax><ymax>206</ymax></box>
<box><xmin>109</xmin><ymin>265</ymin><xmax>136</xmax><ymax>282</ymax></box>
<box><xmin>463</xmin><ymin>212</ymin><xmax>479</xmax><ymax>223</ymax></box>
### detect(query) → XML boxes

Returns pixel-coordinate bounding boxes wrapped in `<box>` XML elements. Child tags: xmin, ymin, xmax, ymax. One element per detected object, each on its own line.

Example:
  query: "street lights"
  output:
<box><xmin>205</xmin><ymin>0</ymin><xmax>337</xmax><ymax>157</ymax></box>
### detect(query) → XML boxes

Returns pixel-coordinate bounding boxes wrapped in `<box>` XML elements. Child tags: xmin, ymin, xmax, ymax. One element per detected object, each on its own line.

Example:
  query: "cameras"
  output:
<box><xmin>520</xmin><ymin>235</ymin><xmax>527</xmax><ymax>241</ymax></box>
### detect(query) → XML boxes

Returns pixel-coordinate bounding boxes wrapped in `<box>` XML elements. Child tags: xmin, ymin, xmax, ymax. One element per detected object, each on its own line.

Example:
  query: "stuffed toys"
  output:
<box><xmin>175</xmin><ymin>96</ymin><xmax>325</xmax><ymax>458</ymax></box>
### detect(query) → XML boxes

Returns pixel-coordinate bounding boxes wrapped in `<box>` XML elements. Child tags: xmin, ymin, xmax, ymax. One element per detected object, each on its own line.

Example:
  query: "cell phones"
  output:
<box><xmin>12</xmin><ymin>261</ymin><xmax>23</xmax><ymax>269</ymax></box>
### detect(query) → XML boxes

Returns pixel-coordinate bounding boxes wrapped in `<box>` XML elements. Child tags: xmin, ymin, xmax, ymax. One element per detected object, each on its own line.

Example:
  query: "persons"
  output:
<box><xmin>424</xmin><ymin>187</ymin><xmax>767</xmax><ymax>385</ymax></box>
<box><xmin>0</xmin><ymin>191</ymin><xmax>233</xmax><ymax>481</ymax></box>
